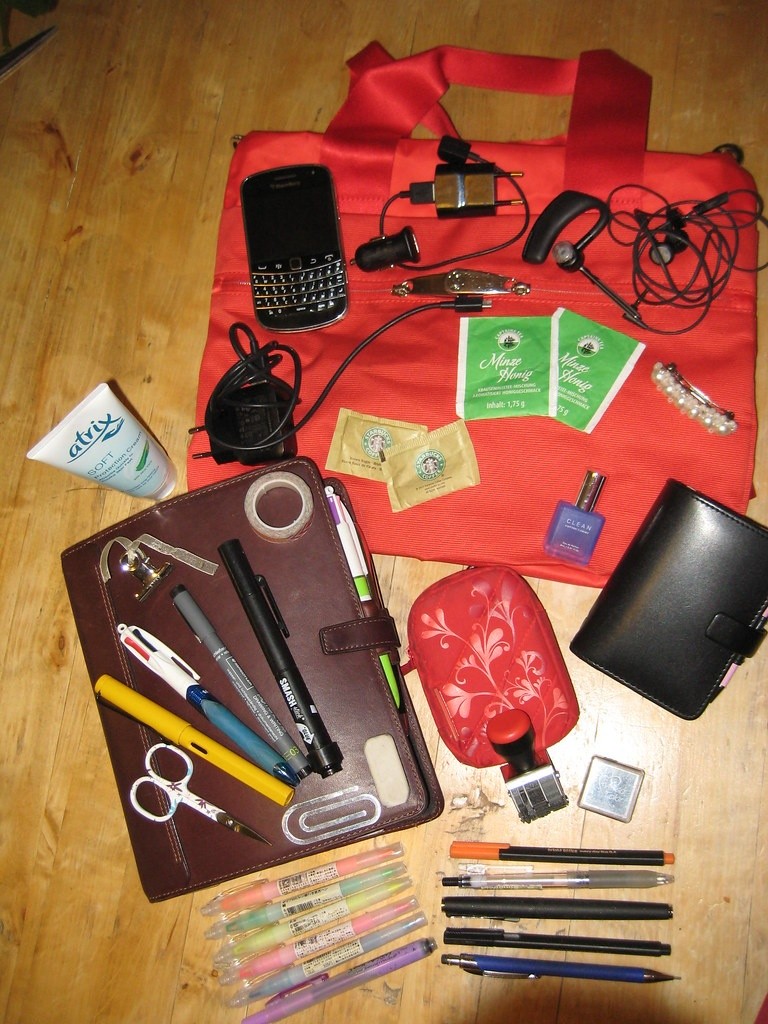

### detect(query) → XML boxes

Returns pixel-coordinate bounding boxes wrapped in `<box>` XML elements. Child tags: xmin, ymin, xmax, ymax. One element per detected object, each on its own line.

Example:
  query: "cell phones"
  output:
<box><xmin>240</xmin><ymin>165</ymin><xmax>350</xmax><ymax>334</ymax></box>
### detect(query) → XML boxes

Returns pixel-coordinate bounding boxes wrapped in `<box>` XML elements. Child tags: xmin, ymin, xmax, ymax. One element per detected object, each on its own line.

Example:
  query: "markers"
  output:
<box><xmin>240</xmin><ymin>936</ymin><xmax>438</xmax><ymax>1024</ymax></box>
<box><xmin>199</xmin><ymin>839</ymin><xmax>428</xmax><ymax>1007</ymax></box>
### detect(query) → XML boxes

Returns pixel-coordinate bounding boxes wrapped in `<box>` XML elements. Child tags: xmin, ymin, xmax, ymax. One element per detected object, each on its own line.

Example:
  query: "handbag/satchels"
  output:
<box><xmin>186</xmin><ymin>40</ymin><xmax>757</xmax><ymax>584</ymax></box>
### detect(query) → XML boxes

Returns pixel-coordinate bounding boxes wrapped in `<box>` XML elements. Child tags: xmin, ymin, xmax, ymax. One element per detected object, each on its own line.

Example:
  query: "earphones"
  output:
<box><xmin>663</xmin><ymin>210</ymin><xmax>690</xmax><ymax>255</ymax></box>
<box><xmin>650</xmin><ymin>236</ymin><xmax>672</xmax><ymax>265</ymax></box>
<box><xmin>552</xmin><ymin>241</ymin><xmax>586</xmax><ymax>272</ymax></box>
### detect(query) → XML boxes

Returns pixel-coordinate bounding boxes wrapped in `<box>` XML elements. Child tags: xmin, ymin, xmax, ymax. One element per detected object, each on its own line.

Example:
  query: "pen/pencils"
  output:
<box><xmin>443</xmin><ymin>926</ymin><xmax>672</xmax><ymax>957</ymax></box>
<box><xmin>450</xmin><ymin>839</ymin><xmax>675</xmax><ymax>867</ymax></box>
<box><xmin>440</xmin><ymin>953</ymin><xmax>682</xmax><ymax>984</ymax></box>
<box><xmin>440</xmin><ymin>896</ymin><xmax>673</xmax><ymax>922</ymax></box>
<box><xmin>442</xmin><ymin>870</ymin><xmax>675</xmax><ymax>889</ymax></box>
<box><xmin>116</xmin><ymin>622</ymin><xmax>300</xmax><ymax>789</ymax></box>
<box><xmin>216</xmin><ymin>539</ymin><xmax>345</xmax><ymax>781</ymax></box>
<box><xmin>92</xmin><ymin>673</ymin><xmax>296</xmax><ymax>809</ymax></box>
<box><xmin>324</xmin><ymin>485</ymin><xmax>400</xmax><ymax>709</ymax></box>
<box><xmin>168</xmin><ymin>583</ymin><xmax>313</xmax><ymax>782</ymax></box>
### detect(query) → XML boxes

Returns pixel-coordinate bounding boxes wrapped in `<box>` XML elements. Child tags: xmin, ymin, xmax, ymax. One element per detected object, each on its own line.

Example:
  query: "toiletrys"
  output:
<box><xmin>26</xmin><ymin>383</ymin><xmax>177</xmax><ymax>501</ymax></box>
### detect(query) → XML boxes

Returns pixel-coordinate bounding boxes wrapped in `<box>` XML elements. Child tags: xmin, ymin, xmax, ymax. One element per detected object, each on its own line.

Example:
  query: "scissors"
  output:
<box><xmin>128</xmin><ymin>742</ymin><xmax>273</xmax><ymax>848</ymax></box>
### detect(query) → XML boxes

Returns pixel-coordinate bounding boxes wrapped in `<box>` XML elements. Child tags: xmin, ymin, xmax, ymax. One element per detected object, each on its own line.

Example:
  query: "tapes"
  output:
<box><xmin>243</xmin><ymin>471</ymin><xmax>314</xmax><ymax>545</ymax></box>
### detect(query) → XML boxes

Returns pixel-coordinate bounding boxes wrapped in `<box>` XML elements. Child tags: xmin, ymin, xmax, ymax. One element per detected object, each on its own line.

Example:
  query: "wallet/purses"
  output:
<box><xmin>406</xmin><ymin>566</ymin><xmax>580</xmax><ymax>769</ymax></box>
